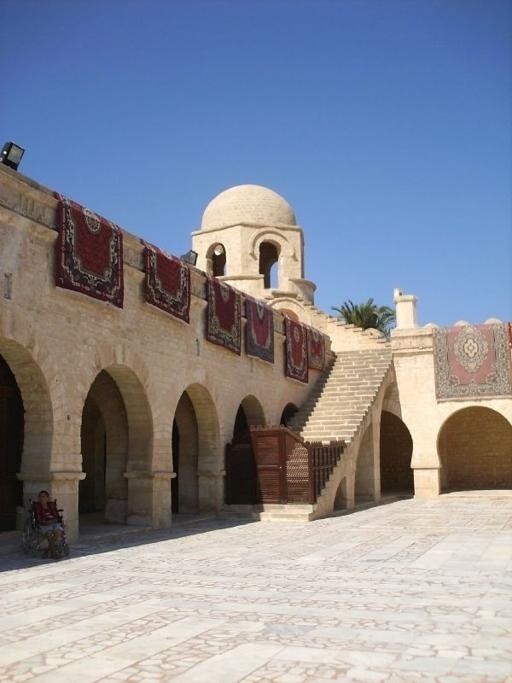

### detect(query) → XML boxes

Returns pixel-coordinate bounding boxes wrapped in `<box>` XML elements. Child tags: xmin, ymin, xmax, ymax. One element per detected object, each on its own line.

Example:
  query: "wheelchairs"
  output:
<box><xmin>22</xmin><ymin>495</ymin><xmax>70</xmax><ymax>559</ymax></box>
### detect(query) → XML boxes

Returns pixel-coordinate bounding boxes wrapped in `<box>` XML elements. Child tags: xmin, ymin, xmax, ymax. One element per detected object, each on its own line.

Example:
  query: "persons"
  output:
<box><xmin>29</xmin><ymin>488</ymin><xmax>65</xmax><ymax>559</ymax></box>
